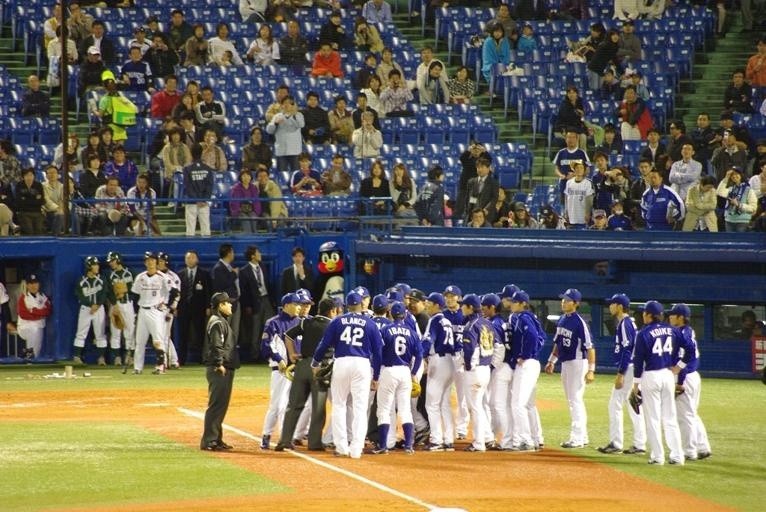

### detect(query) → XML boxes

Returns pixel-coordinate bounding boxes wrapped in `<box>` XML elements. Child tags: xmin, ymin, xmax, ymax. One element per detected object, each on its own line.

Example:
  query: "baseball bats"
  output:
<box><xmin>166</xmin><ymin>326</ymin><xmax>172</xmax><ymax>369</ymax></box>
<box><xmin>122</xmin><ymin>325</ymin><xmax>136</xmax><ymax>373</ymax></box>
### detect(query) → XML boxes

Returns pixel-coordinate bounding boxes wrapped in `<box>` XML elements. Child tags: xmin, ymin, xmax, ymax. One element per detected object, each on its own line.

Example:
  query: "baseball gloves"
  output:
<box><xmin>112</xmin><ymin>313</ymin><xmax>126</xmax><ymax>329</ymax></box>
<box><xmin>316</xmin><ymin>361</ymin><xmax>334</xmax><ymax>389</ymax></box>
<box><xmin>630</xmin><ymin>389</ymin><xmax>642</xmax><ymax>414</ymax></box>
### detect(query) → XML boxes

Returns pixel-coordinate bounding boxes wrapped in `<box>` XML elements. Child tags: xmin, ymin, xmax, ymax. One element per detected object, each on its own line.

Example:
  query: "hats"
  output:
<box><xmin>665</xmin><ymin>303</ymin><xmax>691</xmax><ymax>317</ymax></box>
<box><xmin>391</xmin><ymin>142</ymin><xmax>529</xmax><ymax>221</ymax></box>
<box><xmin>567</xmin><ymin>20</ymin><xmax>665</xmax><ymax>219</ymax></box>
<box><xmin>637</xmin><ymin>300</ymin><xmax>665</xmax><ymax>317</ymax></box>
<box><xmin>558</xmin><ymin>287</ymin><xmax>583</xmax><ymax>304</ymax></box>
<box><xmin>679</xmin><ymin>85</ymin><xmax>766</xmax><ymax>220</ymax></box>
<box><xmin>280</xmin><ymin>282</ymin><xmax>529</xmax><ymax>314</ymax></box>
<box><xmin>87</xmin><ymin>14</ymin><xmax>224</xmax><ymax>217</ymax></box>
<box><xmin>257</xmin><ymin>14</ymin><xmax>444</xmax><ymax>83</ymax></box>
<box><xmin>468</xmin><ymin>16</ymin><xmax>534</xmax><ymax>43</ymax></box>
<box><xmin>604</xmin><ymin>293</ymin><xmax>631</xmax><ymax>310</ymax></box>
<box><xmin>210</xmin><ymin>291</ymin><xmax>237</xmax><ymax>306</ymax></box>
<box><xmin>26</xmin><ymin>273</ymin><xmax>40</xmax><ymax>284</ymax></box>
<box><xmin>235</xmin><ymin>87</ymin><xmax>387</xmax><ymax>226</ymax></box>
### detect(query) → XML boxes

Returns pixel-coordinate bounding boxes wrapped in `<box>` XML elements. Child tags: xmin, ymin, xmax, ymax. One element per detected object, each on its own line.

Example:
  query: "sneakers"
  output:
<box><xmin>668</xmin><ymin>460</ymin><xmax>681</xmax><ymax>465</ymax></box>
<box><xmin>697</xmin><ymin>451</ymin><xmax>712</xmax><ymax>460</ymax></box>
<box><xmin>321</xmin><ymin>421</ymin><xmax>545</xmax><ymax>456</ymax></box>
<box><xmin>647</xmin><ymin>459</ymin><xmax>664</xmax><ymax>466</ymax></box>
<box><xmin>200</xmin><ymin>442</ymin><xmax>234</xmax><ymax>452</ymax></box>
<box><xmin>260</xmin><ymin>434</ymin><xmax>271</xmax><ymax>449</ymax></box>
<box><xmin>560</xmin><ymin>439</ymin><xmax>590</xmax><ymax>449</ymax></box>
<box><xmin>623</xmin><ymin>446</ymin><xmax>646</xmax><ymax>456</ymax></box>
<box><xmin>73</xmin><ymin>354</ymin><xmax>181</xmax><ymax>376</ymax></box>
<box><xmin>274</xmin><ymin>440</ymin><xmax>289</xmax><ymax>451</ymax></box>
<box><xmin>595</xmin><ymin>442</ymin><xmax>623</xmax><ymax>455</ymax></box>
<box><xmin>283</xmin><ymin>442</ymin><xmax>297</xmax><ymax>452</ymax></box>
<box><xmin>307</xmin><ymin>446</ymin><xmax>325</xmax><ymax>452</ymax></box>
<box><xmin>291</xmin><ymin>438</ymin><xmax>304</xmax><ymax>446</ymax></box>
<box><xmin>685</xmin><ymin>454</ymin><xmax>697</xmax><ymax>462</ymax></box>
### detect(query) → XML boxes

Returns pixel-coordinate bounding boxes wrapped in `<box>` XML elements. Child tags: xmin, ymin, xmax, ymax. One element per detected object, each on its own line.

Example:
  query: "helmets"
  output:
<box><xmin>84</xmin><ymin>256</ymin><xmax>101</xmax><ymax>271</ymax></box>
<box><xmin>157</xmin><ymin>252</ymin><xmax>170</xmax><ymax>267</ymax></box>
<box><xmin>105</xmin><ymin>251</ymin><xmax>122</xmax><ymax>265</ymax></box>
<box><xmin>143</xmin><ymin>250</ymin><xmax>158</xmax><ymax>262</ymax></box>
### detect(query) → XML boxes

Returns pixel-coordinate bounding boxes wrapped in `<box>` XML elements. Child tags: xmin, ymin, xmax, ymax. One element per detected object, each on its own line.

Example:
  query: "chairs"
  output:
<box><xmin>437</xmin><ymin>4</ymin><xmax>719</xmax><ymax>152</ymax></box>
<box><xmin>74</xmin><ymin>78</ymin><xmax>531</xmax><ymax>227</ymax></box>
<box><xmin>9</xmin><ymin>3</ymin><xmax>421</xmax><ymax>80</ymax></box>
<box><xmin>2</xmin><ymin>68</ymin><xmax>62</xmax><ymax>182</ymax></box>
<box><xmin>733</xmin><ymin>86</ymin><xmax>766</xmax><ymax>142</ymax></box>
<box><xmin>517</xmin><ymin>184</ymin><xmax>564</xmax><ymax>217</ymax></box>
<box><xmin>606</xmin><ymin>140</ymin><xmax>646</xmax><ymax>174</ymax></box>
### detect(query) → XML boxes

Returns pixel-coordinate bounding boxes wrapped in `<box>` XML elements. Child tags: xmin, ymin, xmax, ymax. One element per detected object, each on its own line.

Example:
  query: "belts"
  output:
<box><xmin>142</xmin><ymin>307</ymin><xmax>151</xmax><ymax>309</ymax></box>
